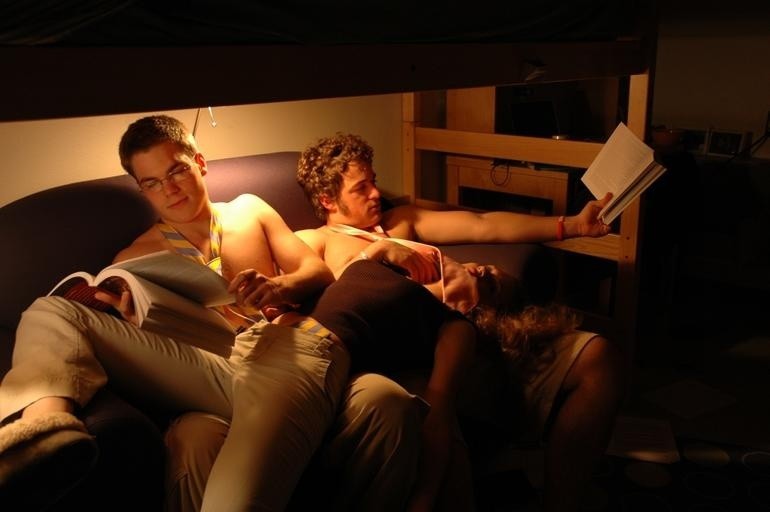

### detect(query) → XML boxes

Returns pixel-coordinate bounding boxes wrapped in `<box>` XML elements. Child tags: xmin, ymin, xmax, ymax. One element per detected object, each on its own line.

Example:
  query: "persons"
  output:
<box><xmin>272</xmin><ymin>128</ymin><xmax>624</xmax><ymax>509</ymax></box>
<box><xmin>0</xmin><ymin>251</ymin><xmax>580</xmax><ymax>512</ymax></box>
<box><xmin>92</xmin><ymin>112</ymin><xmax>433</xmax><ymax>512</ymax></box>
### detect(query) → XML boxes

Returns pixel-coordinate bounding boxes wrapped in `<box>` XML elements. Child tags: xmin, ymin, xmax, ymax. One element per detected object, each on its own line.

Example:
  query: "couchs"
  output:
<box><xmin>0</xmin><ymin>150</ymin><xmax>539</xmax><ymax>511</ymax></box>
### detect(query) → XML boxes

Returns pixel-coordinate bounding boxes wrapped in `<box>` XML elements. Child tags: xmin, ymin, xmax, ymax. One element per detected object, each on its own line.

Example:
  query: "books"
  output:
<box><xmin>46</xmin><ymin>245</ymin><xmax>239</xmax><ymax>361</ymax></box>
<box><xmin>578</xmin><ymin>120</ymin><xmax>667</xmax><ymax>228</ymax></box>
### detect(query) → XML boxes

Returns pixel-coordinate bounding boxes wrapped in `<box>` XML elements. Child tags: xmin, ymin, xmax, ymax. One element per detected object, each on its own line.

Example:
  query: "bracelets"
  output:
<box><xmin>359</xmin><ymin>251</ymin><xmax>369</xmax><ymax>260</ymax></box>
<box><xmin>557</xmin><ymin>216</ymin><xmax>565</xmax><ymax>241</ymax></box>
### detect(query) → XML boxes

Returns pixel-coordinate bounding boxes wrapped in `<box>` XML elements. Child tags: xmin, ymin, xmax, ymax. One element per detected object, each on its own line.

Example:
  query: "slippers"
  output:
<box><xmin>0</xmin><ymin>411</ymin><xmax>110</xmax><ymax>490</ymax></box>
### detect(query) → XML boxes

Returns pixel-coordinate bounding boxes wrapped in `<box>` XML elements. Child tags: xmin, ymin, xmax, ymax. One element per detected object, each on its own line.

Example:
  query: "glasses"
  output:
<box><xmin>140</xmin><ymin>162</ymin><xmax>194</xmax><ymax>194</ymax></box>
